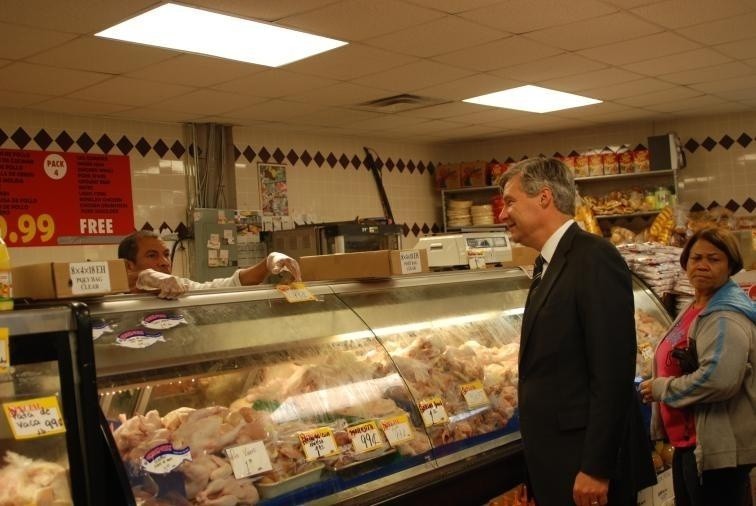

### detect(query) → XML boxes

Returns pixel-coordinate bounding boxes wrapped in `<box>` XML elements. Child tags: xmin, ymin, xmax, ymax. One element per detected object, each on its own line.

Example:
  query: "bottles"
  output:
<box><xmin>0</xmin><ymin>238</ymin><xmax>14</xmax><ymax>311</ymax></box>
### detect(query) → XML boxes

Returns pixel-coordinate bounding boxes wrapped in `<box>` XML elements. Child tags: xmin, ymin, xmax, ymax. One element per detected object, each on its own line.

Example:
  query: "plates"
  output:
<box><xmin>254</xmin><ymin>461</ymin><xmax>326</xmax><ymax>501</ymax></box>
<box><xmin>323</xmin><ymin>447</ymin><xmax>397</xmax><ymax>478</ymax></box>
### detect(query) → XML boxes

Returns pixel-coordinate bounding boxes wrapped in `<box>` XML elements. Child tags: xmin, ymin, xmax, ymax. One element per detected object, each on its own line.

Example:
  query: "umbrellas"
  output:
<box><xmin>665</xmin><ymin>337</ymin><xmax>701</xmax><ymax>375</ymax></box>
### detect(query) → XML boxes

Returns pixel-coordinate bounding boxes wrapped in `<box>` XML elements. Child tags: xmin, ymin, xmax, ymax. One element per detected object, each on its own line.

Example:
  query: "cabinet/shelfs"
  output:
<box><xmin>0</xmin><ymin>262</ymin><xmax>675</xmax><ymax>506</ymax></box>
<box><xmin>438</xmin><ymin>135</ymin><xmax>682</xmax><ymax>236</ymax></box>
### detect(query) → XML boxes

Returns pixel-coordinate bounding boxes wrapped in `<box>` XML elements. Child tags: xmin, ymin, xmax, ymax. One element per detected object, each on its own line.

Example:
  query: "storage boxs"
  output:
<box><xmin>636</xmin><ymin>463</ymin><xmax>675</xmax><ymax>502</ymax></box>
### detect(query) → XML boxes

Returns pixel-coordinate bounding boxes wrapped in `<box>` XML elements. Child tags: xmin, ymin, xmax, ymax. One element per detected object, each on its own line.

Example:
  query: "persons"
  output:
<box><xmin>116</xmin><ymin>230</ymin><xmax>302</xmax><ymax>300</ymax></box>
<box><xmin>638</xmin><ymin>224</ymin><xmax>756</xmax><ymax>506</ymax></box>
<box><xmin>498</xmin><ymin>157</ymin><xmax>638</xmax><ymax>506</ymax></box>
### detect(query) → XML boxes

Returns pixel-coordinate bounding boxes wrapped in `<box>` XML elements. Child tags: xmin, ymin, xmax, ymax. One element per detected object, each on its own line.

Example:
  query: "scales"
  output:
<box><xmin>413</xmin><ymin>227</ymin><xmax>513</xmax><ymax>271</ymax></box>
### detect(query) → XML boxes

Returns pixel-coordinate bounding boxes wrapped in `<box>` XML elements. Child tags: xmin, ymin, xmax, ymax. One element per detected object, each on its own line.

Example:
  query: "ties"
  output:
<box><xmin>526</xmin><ymin>254</ymin><xmax>547</xmax><ymax>303</ymax></box>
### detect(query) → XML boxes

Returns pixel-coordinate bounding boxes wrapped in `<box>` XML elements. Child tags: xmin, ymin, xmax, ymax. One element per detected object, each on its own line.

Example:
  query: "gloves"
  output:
<box><xmin>265</xmin><ymin>250</ymin><xmax>303</xmax><ymax>285</ymax></box>
<box><xmin>135</xmin><ymin>268</ymin><xmax>188</xmax><ymax>301</ymax></box>
